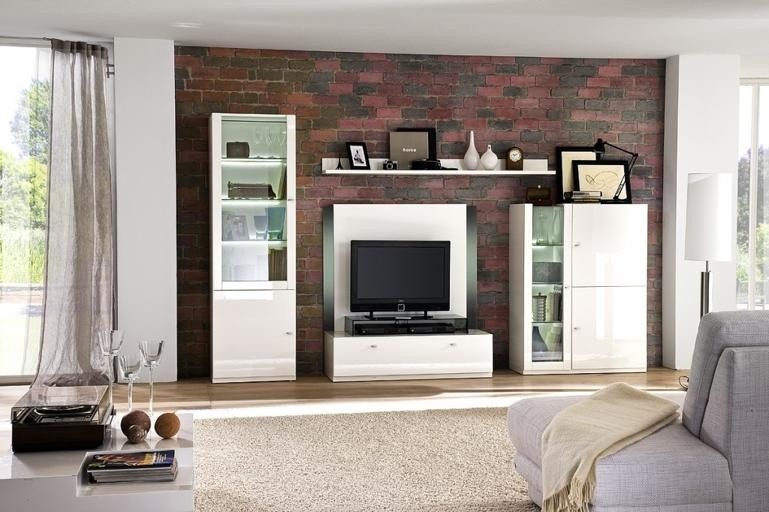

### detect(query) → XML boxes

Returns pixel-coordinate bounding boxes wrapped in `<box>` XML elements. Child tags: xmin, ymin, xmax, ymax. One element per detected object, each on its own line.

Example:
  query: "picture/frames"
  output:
<box><xmin>345</xmin><ymin>140</ymin><xmax>369</xmax><ymax>169</ymax></box>
<box><xmin>572</xmin><ymin>159</ymin><xmax>632</xmax><ymax>203</ymax></box>
<box><xmin>555</xmin><ymin>146</ymin><xmax>601</xmax><ymax>202</ymax></box>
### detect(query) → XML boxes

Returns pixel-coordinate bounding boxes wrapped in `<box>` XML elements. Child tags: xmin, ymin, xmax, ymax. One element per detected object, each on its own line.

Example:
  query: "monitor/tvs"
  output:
<box><xmin>351</xmin><ymin>240</ymin><xmax>450</xmax><ymax>320</ymax></box>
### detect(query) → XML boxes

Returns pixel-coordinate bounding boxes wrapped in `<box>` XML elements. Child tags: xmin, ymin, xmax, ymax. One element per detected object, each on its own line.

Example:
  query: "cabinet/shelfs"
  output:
<box><xmin>209</xmin><ymin>110</ymin><xmax>298</xmax><ymax>385</ymax></box>
<box><xmin>507</xmin><ymin>201</ymin><xmax>648</xmax><ymax>375</ymax></box>
<box><xmin>325</xmin><ymin>326</ymin><xmax>495</xmax><ymax>381</ymax></box>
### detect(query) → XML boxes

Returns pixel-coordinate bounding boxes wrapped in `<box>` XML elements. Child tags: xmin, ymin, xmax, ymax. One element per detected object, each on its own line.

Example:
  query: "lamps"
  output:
<box><xmin>592</xmin><ymin>137</ymin><xmax>639</xmax><ymax>202</ymax></box>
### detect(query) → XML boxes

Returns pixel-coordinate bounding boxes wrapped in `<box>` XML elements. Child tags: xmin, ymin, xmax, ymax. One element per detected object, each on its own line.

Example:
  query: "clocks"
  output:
<box><xmin>506</xmin><ymin>147</ymin><xmax>524</xmax><ymax>171</ymax></box>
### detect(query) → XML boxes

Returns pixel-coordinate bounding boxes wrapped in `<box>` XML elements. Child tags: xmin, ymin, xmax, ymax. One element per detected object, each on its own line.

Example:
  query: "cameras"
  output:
<box><xmin>383</xmin><ymin>161</ymin><xmax>397</xmax><ymax>170</ymax></box>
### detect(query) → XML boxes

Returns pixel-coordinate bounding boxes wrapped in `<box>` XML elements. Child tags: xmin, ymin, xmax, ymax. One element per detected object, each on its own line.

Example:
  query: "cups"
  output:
<box><xmin>253</xmin><ymin>215</ymin><xmax>268</xmax><ymax>240</ymax></box>
<box><xmin>230</xmin><ymin>215</ymin><xmax>250</xmax><ymax>240</ymax></box>
<box><xmin>222</xmin><ymin>210</ymin><xmax>235</xmax><ymax>241</ymax></box>
<box><xmin>95</xmin><ymin>328</ymin><xmax>125</xmax><ymax>416</ymax></box>
<box><xmin>139</xmin><ymin>336</ymin><xmax>165</xmax><ymax>417</ymax></box>
<box><xmin>264</xmin><ymin>207</ymin><xmax>285</xmax><ymax>240</ymax></box>
<box><xmin>277</xmin><ymin>128</ymin><xmax>287</xmax><ymax>157</ymax></box>
<box><xmin>268</xmin><ymin>126</ymin><xmax>277</xmax><ymax>157</ymax></box>
<box><xmin>255</xmin><ymin>126</ymin><xmax>267</xmax><ymax>157</ymax></box>
<box><xmin>115</xmin><ymin>348</ymin><xmax>144</xmax><ymax>414</ymax></box>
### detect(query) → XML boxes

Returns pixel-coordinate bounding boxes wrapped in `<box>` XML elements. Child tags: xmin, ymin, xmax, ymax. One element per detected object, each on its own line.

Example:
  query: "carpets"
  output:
<box><xmin>189</xmin><ymin>407</ymin><xmax>542</xmax><ymax>512</ymax></box>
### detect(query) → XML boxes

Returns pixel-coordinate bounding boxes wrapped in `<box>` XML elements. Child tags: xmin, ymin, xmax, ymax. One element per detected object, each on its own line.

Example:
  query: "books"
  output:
<box><xmin>86</xmin><ymin>450</ymin><xmax>179</xmax><ymax>482</ymax></box>
<box><xmin>268</xmin><ymin>246</ymin><xmax>287</xmax><ymax>281</ymax></box>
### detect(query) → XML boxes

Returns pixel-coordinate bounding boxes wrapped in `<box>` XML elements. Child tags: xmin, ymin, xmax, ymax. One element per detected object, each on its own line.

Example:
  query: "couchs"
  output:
<box><xmin>509</xmin><ymin>310</ymin><xmax>769</xmax><ymax>512</ymax></box>
